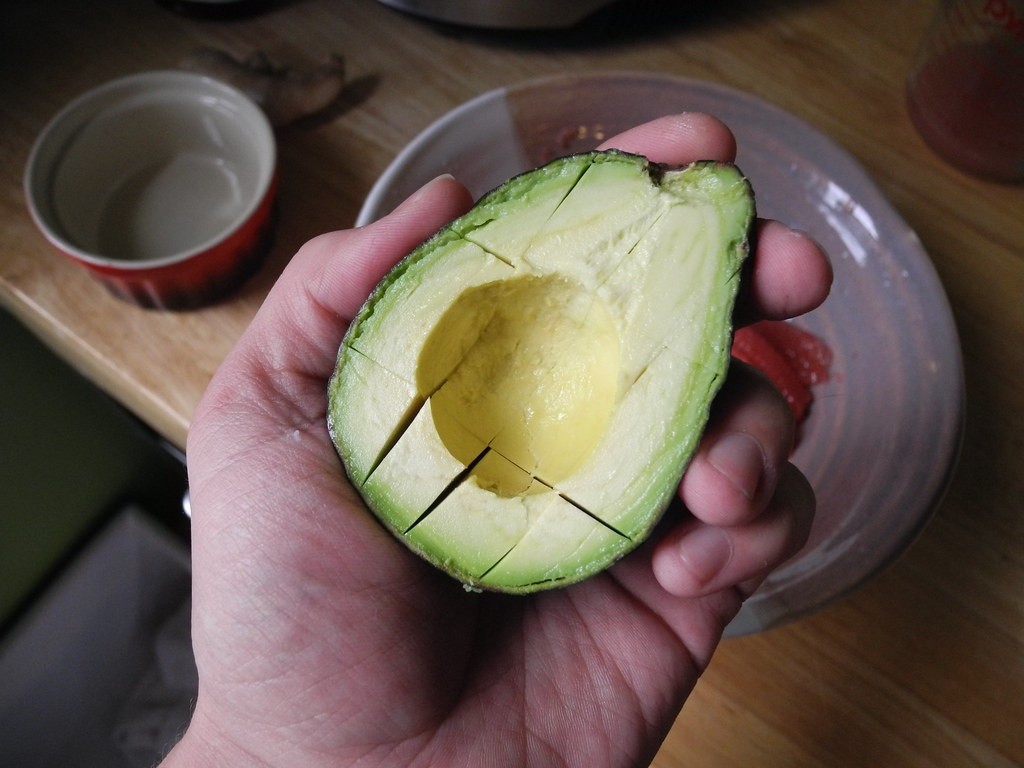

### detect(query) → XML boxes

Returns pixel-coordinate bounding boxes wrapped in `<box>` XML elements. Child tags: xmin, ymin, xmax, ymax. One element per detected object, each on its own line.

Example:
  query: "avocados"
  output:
<box><xmin>323</xmin><ymin>151</ymin><xmax>757</xmax><ymax>594</ymax></box>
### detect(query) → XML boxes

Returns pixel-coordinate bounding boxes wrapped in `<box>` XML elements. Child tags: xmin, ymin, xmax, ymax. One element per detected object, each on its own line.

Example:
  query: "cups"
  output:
<box><xmin>904</xmin><ymin>0</ymin><xmax>1024</xmax><ymax>185</ymax></box>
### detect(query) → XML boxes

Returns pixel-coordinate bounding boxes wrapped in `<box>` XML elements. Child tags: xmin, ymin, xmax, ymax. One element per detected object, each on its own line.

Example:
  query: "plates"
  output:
<box><xmin>356</xmin><ymin>71</ymin><xmax>966</xmax><ymax>638</ymax></box>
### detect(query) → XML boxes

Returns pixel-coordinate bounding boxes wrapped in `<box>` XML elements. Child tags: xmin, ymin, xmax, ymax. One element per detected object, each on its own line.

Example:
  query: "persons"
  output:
<box><xmin>160</xmin><ymin>111</ymin><xmax>834</xmax><ymax>768</ymax></box>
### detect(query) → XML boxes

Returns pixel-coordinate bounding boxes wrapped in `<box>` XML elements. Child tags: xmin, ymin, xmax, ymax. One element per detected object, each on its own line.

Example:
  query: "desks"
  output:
<box><xmin>0</xmin><ymin>0</ymin><xmax>1024</xmax><ymax>768</ymax></box>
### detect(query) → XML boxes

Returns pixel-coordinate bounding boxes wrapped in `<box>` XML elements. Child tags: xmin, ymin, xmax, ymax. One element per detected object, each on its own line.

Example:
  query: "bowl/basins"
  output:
<box><xmin>26</xmin><ymin>69</ymin><xmax>281</xmax><ymax>311</ymax></box>
